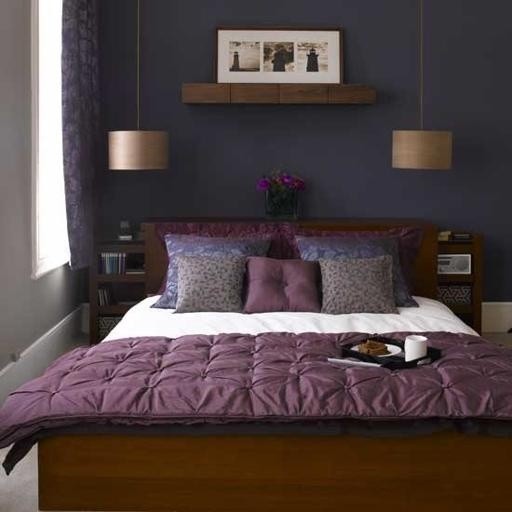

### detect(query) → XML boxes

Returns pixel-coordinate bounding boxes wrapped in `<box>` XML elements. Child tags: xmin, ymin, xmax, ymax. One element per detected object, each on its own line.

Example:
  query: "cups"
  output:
<box><xmin>404</xmin><ymin>334</ymin><xmax>430</xmax><ymax>362</ymax></box>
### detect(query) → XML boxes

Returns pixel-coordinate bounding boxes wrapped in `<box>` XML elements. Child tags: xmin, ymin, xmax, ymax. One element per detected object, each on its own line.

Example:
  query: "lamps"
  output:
<box><xmin>390</xmin><ymin>0</ymin><xmax>454</xmax><ymax>170</ymax></box>
<box><xmin>107</xmin><ymin>0</ymin><xmax>169</xmax><ymax>171</ymax></box>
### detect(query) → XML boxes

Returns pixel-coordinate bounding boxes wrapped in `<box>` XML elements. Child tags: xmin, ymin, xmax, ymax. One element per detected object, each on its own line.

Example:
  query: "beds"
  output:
<box><xmin>38</xmin><ymin>219</ymin><xmax>512</xmax><ymax>512</ymax></box>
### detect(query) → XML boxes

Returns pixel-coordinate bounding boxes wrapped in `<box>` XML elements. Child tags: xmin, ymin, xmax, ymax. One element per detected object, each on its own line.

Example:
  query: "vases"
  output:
<box><xmin>263</xmin><ymin>190</ymin><xmax>297</xmax><ymax>221</ymax></box>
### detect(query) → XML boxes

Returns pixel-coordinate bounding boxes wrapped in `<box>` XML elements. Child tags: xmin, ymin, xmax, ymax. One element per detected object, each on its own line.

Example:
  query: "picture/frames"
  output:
<box><xmin>215</xmin><ymin>25</ymin><xmax>344</xmax><ymax>86</ymax></box>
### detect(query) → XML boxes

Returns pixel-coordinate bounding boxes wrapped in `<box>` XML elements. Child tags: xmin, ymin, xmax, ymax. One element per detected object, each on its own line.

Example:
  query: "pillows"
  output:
<box><xmin>172</xmin><ymin>255</ymin><xmax>249</xmax><ymax>314</ymax></box>
<box><xmin>154</xmin><ymin>224</ymin><xmax>288</xmax><ymax>296</ymax></box>
<box><xmin>288</xmin><ymin>221</ymin><xmax>424</xmax><ymax>296</ymax></box>
<box><xmin>295</xmin><ymin>235</ymin><xmax>419</xmax><ymax>307</ymax></box>
<box><xmin>243</xmin><ymin>256</ymin><xmax>320</xmax><ymax>314</ymax></box>
<box><xmin>319</xmin><ymin>254</ymin><xmax>399</xmax><ymax>314</ymax></box>
<box><xmin>149</xmin><ymin>231</ymin><xmax>273</xmax><ymax>309</ymax></box>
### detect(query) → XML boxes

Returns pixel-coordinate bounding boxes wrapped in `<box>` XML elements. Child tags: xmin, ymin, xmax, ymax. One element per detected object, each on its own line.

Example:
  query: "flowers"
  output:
<box><xmin>255</xmin><ymin>167</ymin><xmax>305</xmax><ymax>213</ymax></box>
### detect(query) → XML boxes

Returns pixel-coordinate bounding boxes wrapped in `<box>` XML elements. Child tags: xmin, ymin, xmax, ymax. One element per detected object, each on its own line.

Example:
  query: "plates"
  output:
<box><xmin>350</xmin><ymin>342</ymin><xmax>402</xmax><ymax>359</ymax></box>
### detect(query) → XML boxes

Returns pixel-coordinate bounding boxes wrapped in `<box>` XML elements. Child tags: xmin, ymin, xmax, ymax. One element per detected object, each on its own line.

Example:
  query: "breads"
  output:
<box><xmin>358</xmin><ymin>339</ymin><xmax>388</xmax><ymax>356</ymax></box>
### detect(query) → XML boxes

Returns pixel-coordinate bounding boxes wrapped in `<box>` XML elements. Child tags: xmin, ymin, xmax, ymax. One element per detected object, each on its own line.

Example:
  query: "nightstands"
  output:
<box><xmin>436</xmin><ymin>233</ymin><xmax>485</xmax><ymax>336</ymax></box>
<box><xmin>89</xmin><ymin>241</ymin><xmax>145</xmax><ymax>346</ymax></box>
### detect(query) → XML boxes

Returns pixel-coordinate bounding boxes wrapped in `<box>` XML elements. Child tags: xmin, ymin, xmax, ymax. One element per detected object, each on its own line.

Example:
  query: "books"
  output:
<box><xmin>98</xmin><ymin>287</ymin><xmax>113</xmax><ymax>307</ymax></box>
<box><xmin>100</xmin><ymin>251</ymin><xmax>146</xmax><ymax>274</ymax></box>
